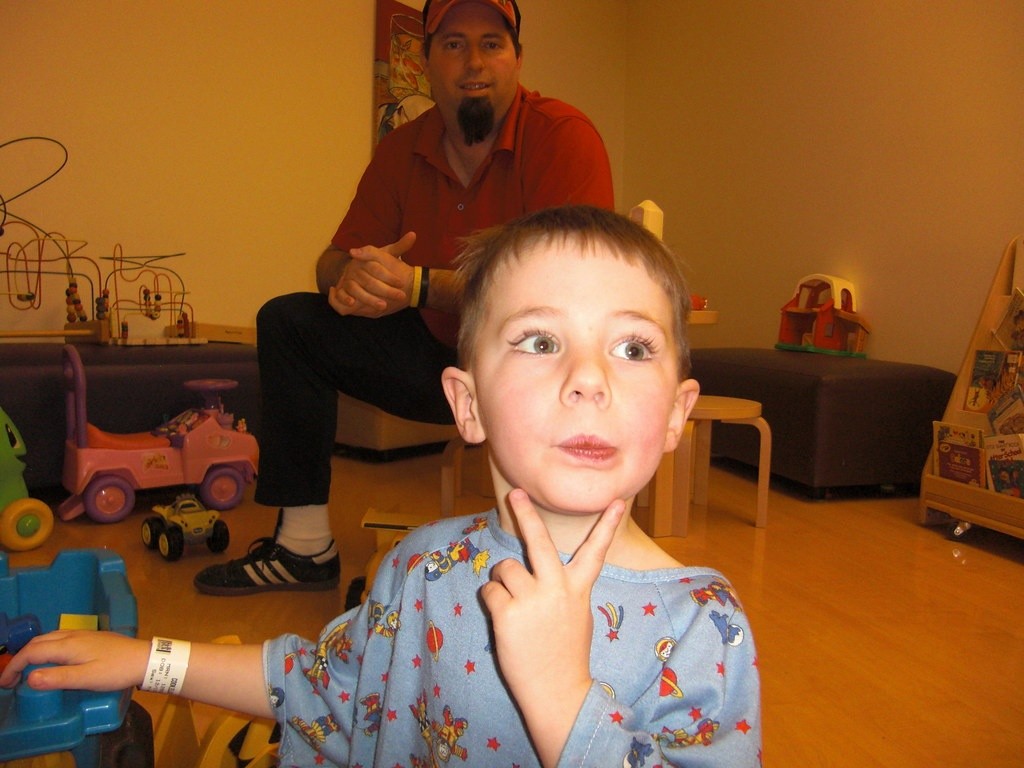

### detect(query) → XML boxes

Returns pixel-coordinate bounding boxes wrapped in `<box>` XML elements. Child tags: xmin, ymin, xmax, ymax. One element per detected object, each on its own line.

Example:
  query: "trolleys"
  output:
<box><xmin>56</xmin><ymin>344</ymin><xmax>260</xmax><ymax>522</ymax></box>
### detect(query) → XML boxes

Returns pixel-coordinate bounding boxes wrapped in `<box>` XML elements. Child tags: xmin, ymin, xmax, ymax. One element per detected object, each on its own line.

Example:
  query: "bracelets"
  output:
<box><xmin>139</xmin><ymin>635</ymin><xmax>191</xmax><ymax>696</ymax></box>
<box><xmin>410</xmin><ymin>266</ymin><xmax>430</xmax><ymax>309</ymax></box>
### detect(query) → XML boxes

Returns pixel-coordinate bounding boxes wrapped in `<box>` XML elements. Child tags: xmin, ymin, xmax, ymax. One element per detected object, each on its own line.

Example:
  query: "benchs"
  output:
<box><xmin>689</xmin><ymin>347</ymin><xmax>959</xmax><ymax>500</ymax></box>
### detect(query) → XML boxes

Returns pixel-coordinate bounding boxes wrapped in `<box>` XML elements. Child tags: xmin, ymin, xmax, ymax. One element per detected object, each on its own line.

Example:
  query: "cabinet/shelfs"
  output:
<box><xmin>920</xmin><ymin>232</ymin><xmax>1024</xmax><ymax>540</ymax></box>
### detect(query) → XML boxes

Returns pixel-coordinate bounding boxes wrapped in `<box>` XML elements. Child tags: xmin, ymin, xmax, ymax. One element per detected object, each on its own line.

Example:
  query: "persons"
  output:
<box><xmin>0</xmin><ymin>203</ymin><xmax>762</xmax><ymax>768</ymax></box>
<box><xmin>193</xmin><ymin>0</ymin><xmax>614</xmax><ymax>599</ymax></box>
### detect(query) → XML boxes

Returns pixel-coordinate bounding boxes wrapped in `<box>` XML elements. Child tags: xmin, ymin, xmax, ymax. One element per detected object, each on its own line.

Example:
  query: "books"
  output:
<box><xmin>932</xmin><ymin>287</ymin><xmax>1024</xmax><ymax>500</ymax></box>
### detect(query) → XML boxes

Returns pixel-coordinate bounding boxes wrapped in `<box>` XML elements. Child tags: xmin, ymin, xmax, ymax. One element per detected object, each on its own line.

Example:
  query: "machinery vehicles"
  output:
<box><xmin>142</xmin><ymin>494</ymin><xmax>229</xmax><ymax>560</ymax></box>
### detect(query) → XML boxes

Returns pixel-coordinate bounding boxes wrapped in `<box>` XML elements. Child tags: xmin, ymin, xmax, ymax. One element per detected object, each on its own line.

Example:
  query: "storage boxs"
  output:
<box><xmin>164</xmin><ymin>322</ymin><xmax>257</xmax><ymax>345</ymax></box>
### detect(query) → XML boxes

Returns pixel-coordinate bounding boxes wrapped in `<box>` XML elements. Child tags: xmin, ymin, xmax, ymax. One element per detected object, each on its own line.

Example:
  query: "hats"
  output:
<box><xmin>422</xmin><ymin>0</ymin><xmax>520</xmax><ymax>44</ymax></box>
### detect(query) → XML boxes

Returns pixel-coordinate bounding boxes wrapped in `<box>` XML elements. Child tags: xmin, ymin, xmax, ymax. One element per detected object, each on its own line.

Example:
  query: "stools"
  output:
<box><xmin>637</xmin><ymin>396</ymin><xmax>773</xmax><ymax>529</ymax></box>
<box><xmin>448</xmin><ymin>422</ymin><xmax>494</xmax><ymax>497</ymax></box>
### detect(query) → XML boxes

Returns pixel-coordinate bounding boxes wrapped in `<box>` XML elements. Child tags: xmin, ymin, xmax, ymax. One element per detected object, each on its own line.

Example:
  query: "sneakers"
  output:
<box><xmin>193</xmin><ymin>533</ymin><xmax>341</xmax><ymax>593</ymax></box>
